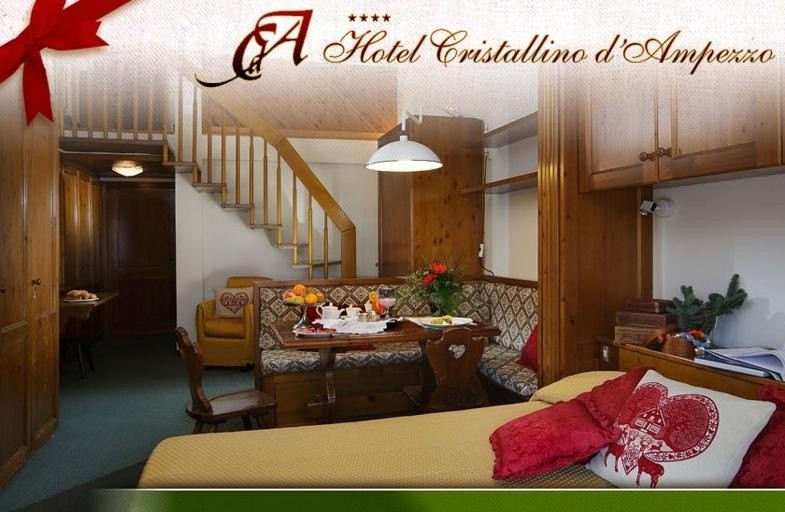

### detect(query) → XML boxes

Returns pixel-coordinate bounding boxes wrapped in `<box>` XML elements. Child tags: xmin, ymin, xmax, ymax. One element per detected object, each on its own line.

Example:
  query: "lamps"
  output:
<box><xmin>363</xmin><ymin>102</ymin><xmax>461</xmax><ymax>174</ymax></box>
<box><xmin>112</xmin><ymin>159</ymin><xmax>144</xmax><ymax>177</ymax></box>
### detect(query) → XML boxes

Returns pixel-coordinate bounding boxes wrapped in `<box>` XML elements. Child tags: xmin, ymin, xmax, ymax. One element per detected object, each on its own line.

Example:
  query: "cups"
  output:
<box><xmin>326</xmin><ymin>305</ymin><xmax>380</xmax><ymax>328</ymax></box>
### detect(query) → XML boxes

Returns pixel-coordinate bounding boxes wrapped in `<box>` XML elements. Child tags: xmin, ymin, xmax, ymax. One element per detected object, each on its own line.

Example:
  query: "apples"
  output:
<box><xmin>285</xmin><ymin>291</ymin><xmax>296</xmax><ymax>298</ymax></box>
<box><xmin>293</xmin><ymin>284</ymin><xmax>305</xmax><ymax>296</ymax></box>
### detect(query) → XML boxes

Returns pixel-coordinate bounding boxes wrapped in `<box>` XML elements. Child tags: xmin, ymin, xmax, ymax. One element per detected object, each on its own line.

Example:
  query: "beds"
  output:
<box><xmin>127</xmin><ymin>338</ymin><xmax>785</xmax><ymax>512</ymax></box>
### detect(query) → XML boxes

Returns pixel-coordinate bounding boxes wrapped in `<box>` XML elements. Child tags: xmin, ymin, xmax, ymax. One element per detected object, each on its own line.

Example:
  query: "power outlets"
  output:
<box><xmin>478</xmin><ymin>242</ymin><xmax>485</xmax><ymax>260</ymax></box>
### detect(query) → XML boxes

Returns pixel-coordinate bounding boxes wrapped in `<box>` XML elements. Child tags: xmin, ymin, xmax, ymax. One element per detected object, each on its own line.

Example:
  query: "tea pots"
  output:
<box><xmin>316</xmin><ymin>303</ymin><xmax>345</xmax><ymax>320</ymax></box>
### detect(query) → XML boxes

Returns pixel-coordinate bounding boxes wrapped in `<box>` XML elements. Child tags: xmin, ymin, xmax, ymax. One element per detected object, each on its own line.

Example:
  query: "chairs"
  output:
<box><xmin>175</xmin><ymin>327</ymin><xmax>279</xmax><ymax>436</ymax></box>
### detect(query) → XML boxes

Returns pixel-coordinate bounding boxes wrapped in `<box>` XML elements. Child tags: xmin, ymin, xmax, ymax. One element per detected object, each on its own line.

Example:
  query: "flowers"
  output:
<box><xmin>393</xmin><ymin>259</ymin><xmax>470</xmax><ymax>317</ymax></box>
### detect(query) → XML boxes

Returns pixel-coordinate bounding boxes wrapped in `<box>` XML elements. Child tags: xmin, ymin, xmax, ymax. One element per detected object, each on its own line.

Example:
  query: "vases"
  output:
<box><xmin>428</xmin><ymin>296</ymin><xmax>443</xmax><ymax>316</ymax></box>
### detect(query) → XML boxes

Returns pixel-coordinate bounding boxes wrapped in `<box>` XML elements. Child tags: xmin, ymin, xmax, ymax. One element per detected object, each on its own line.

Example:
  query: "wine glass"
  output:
<box><xmin>379</xmin><ymin>287</ymin><xmax>397</xmax><ymax>321</ymax></box>
<box><xmin>281</xmin><ymin>301</ymin><xmax>326</xmax><ymax>330</ymax></box>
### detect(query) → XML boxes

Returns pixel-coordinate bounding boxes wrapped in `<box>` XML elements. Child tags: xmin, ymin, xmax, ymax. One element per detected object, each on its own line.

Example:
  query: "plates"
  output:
<box><xmin>421</xmin><ymin>317</ymin><xmax>473</xmax><ymax>327</ymax></box>
<box><xmin>293</xmin><ymin>330</ymin><xmax>341</xmax><ymax>337</ymax></box>
<box><xmin>64</xmin><ymin>298</ymin><xmax>99</xmax><ymax>302</ymax></box>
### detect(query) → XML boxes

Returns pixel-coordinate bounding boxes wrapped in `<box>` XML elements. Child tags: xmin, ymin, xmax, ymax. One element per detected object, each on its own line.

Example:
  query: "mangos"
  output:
<box><xmin>288</xmin><ymin>296</ymin><xmax>304</xmax><ymax>304</ymax></box>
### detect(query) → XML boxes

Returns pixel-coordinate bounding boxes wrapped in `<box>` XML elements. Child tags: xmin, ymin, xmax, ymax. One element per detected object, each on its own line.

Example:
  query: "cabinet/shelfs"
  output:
<box><xmin>593</xmin><ymin>336</ymin><xmax>784</xmax><ymax>399</ymax></box>
<box><xmin>25</xmin><ymin>92</ymin><xmax>61</xmax><ymax>456</ymax></box>
<box><xmin>375</xmin><ymin>114</ymin><xmax>486</xmax><ymax>276</ymax></box>
<box><xmin>0</xmin><ymin>65</ymin><xmax>26</xmax><ymax>486</ymax></box>
<box><xmin>579</xmin><ymin>56</ymin><xmax>784</xmax><ymax>193</ymax></box>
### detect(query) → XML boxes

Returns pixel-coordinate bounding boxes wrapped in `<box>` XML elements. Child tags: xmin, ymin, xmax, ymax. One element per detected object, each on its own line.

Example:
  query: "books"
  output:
<box><xmin>694</xmin><ymin>345</ymin><xmax>784</xmax><ymax>382</ymax></box>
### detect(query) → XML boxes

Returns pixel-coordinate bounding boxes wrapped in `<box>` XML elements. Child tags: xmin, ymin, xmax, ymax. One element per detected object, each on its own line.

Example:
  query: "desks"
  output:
<box><xmin>58</xmin><ymin>289</ymin><xmax>122</xmax><ymax>380</ymax></box>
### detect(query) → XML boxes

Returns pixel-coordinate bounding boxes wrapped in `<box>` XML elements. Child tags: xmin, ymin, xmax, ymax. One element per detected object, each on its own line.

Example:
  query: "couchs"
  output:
<box><xmin>194</xmin><ymin>275</ymin><xmax>274</xmax><ymax>368</ymax></box>
<box><xmin>251</xmin><ymin>273</ymin><xmax>538</xmax><ymax>429</ymax></box>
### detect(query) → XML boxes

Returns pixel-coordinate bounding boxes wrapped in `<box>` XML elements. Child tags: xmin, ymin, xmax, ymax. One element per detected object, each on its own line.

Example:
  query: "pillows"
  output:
<box><xmin>587</xmin><ymin>368</ymin><xmax>777</xmax><ymax>489</ymax></box>
<box><xmin>581</xmin><ymin>367</ymin><xmax>660</xmax><ymax>423</ymax></box>
<box><xmin>211</xmin><ymin>285</ymin><xmax>253</xmax><ymax>319</ymax></box>
<box><xmin>516</xmin><ymin>323</ymin><xmax>538</xmax><ymax>371</ymax></box>
<box><xmin>731</xmin><ymin>381</ymin><xmax>785</xmax><ymax>487</ymax></box>
<box><xmin>486</xmin><ymin>391</ymin><xmax>623</xmax><ymax>480</ymax></box>
